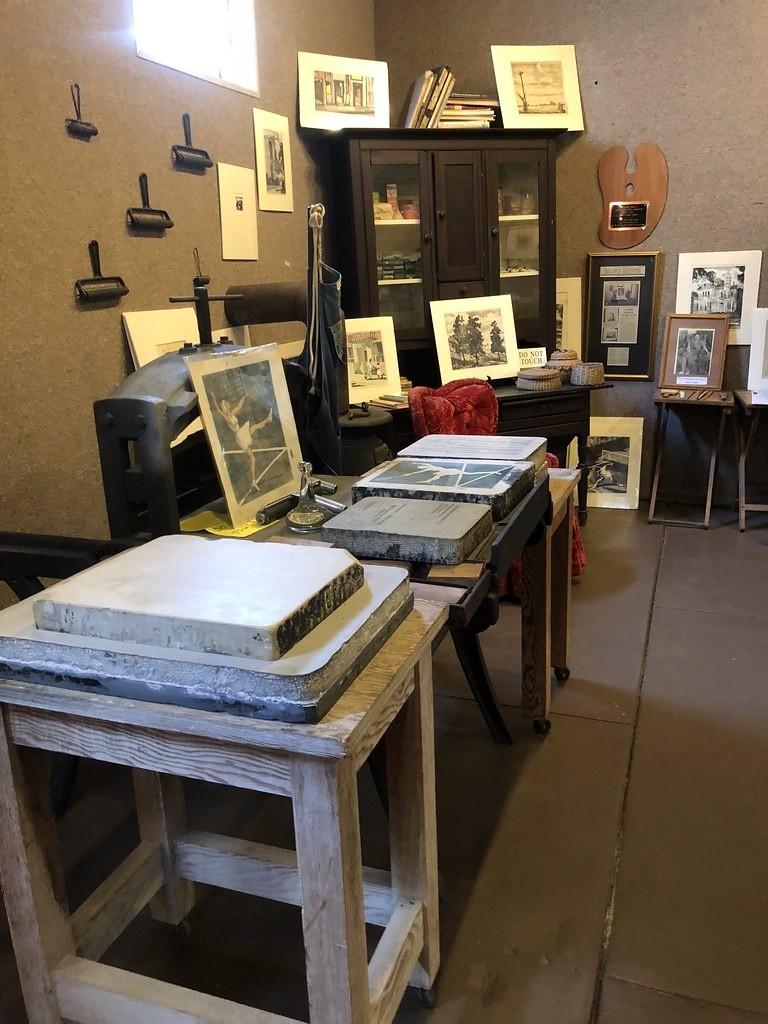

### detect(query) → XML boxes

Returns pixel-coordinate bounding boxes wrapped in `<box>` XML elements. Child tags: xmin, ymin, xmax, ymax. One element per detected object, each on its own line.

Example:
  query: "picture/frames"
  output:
<box><xmin>582</xmin><ymin>251</ymin><xmax>661</xmax><ymax>381</ymax></box>
<box><xmin>187</xmin><ymin>349</ymin><xmax>306</xmax><ymax>526</ymax></box>
<box><xmin>658</xmin><ymin>313</ymin><xmax>731</xmax><ymax>391</ymax></box>
<box><xmin>429</xmin><ymin>293</ymin><xmax>522</xmax><ymax>386</ymax></box>
<box><xmin>344</xmin><ymin>316</ymin><xmax>403</xmax><ymax>404</ymax></box>
<box><xmin>296</xmin><ymin>52</ymin><xmax>390</xmax><ymax>130</ymax></box>
<box><xmin>491</xmin><ymin>44</ymin><xmax>585</xmax><ymax>132</ymax></box>
<box><xmin>570</xmin><ymin>416</ymin><xmax>646</xmax><ymax>509</ymax></box>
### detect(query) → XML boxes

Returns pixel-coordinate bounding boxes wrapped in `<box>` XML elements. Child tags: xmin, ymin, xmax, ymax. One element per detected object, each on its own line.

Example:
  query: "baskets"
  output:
<box><xmin>571</xmin><ymin>362</ymin><xmax>605</xmax><ymax>386</ymax></box>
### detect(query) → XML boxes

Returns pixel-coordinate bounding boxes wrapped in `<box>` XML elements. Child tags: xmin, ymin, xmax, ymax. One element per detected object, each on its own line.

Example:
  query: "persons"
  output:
<box><xmin>608</xmin><ymin>312</ymin><xmax>616</xmax><ymax>321</ymax></box>
<box><xmin>277</xmin><ymin>143</ymin><xmax>285</xmax><ymax>194</ymax></box>
<box><xmin>209</xmin><ymin>387</ymin><xmax>273</xmax><ymax>491</ymax></box>
<box><xmin>625</xmin><ymin>288</ymin><xmax>631</xmax><ymax>305</ymax></box>
<box><xmin>679</xmin><ymin>332</ymin><xmax>709</xmax><ymax>374</ymax></box>
<box><xmin>364</xmin><ymin>358</ymin><xmax>383</xmax><ymax>380</ymax></box>
<box><xmin>591</xmin><ymin>463</ymin><xmax>623</xmax><ymax>489</ymax></box>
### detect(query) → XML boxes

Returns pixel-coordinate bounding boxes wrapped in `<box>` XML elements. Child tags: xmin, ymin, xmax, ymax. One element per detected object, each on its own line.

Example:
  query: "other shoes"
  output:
<box><xmin>685</xmin><ymin>369</ymin><xmax>691</xmax><ymax>375</ymax></box>
<box><xmin>678</xmin><ymin>371</ymin><xmax>685</xmax><ymax>374</ymax></box>
<box><xmin>251</xmin><ymin>484</ymin><xmax>261</xmax><ymax>492</ymax></box>
<box><xmin>619</xmin><ymin>484</ymin><xmax>624</xmax><ymax>486</ymax></box>
<box><xmin>592</xmin><ymin>486</ymin><xmax>598</xmax><ymax>489</ymax></box>
<box><xmin>268</xmin><ymin>408</ymin><xmax>272</xmax><ymax>422</ymax></box>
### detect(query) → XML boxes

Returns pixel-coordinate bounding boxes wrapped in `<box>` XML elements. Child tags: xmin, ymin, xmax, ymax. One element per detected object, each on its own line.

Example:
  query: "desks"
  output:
<box><xmin>337</xmin><ymin>379</ymin><xmax>616</xmax><ymax>527</ymax></box>
<box><xmin>518</xmin><ymin>469</ymin><xmax>581</xmax><ymax>735</ymax></box>
<box><xmin>732</xmin><ymin>390</ymin><xmax>768</xmax><ymax>532</ymax></box>
<box><xmin>0</xmin><ymin>576</ymin><xmax>451</xmax><ymax>1024</ymax></box>
<box><xmin>646</xmin><ymin>387</ymin><xmax>734</xmax><ymax>529</ymax></box>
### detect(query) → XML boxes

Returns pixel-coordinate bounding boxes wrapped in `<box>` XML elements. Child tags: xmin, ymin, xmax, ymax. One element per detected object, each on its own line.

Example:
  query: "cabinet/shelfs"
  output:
<box><xmin>329</xmin><ymin>126</ymin><xmax>569</xmax><ymax>361</ymax></box>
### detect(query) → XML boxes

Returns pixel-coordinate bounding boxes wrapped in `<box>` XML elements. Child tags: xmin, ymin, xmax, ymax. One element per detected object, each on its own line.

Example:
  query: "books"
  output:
<box><xmin>406</xmin><ymin>62</ymin><xmax>500</xmax><ymax>127</ymax></box>
<box><xmin>369</xmin><ymin>377</ymin><xmax>414</xmax><ymax>410</ymax></box>
